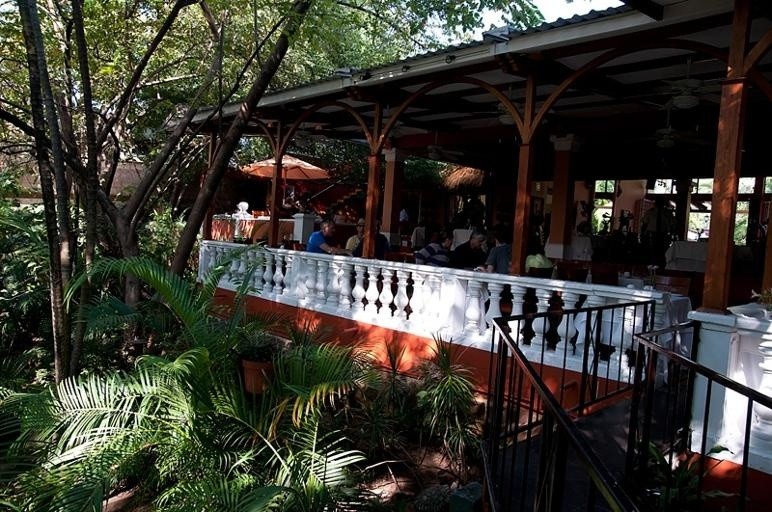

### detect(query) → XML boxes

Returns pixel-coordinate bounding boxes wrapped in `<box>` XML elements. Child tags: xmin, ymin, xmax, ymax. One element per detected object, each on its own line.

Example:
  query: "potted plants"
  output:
<box><xmin>242</xmin><ymin>358</ymin><xmax>276</xmax><ymax>393</ymax></box>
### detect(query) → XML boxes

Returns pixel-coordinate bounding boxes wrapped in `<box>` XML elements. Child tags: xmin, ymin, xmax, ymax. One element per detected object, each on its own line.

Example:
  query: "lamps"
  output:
<box><xmin>549</xmin><ymin>133</ymin><xmax>584</xmax><ymax>153</ymax></box>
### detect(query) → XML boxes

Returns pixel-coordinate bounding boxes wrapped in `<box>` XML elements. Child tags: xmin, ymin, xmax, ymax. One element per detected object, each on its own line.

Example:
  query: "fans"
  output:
<box><xmin>469</xmin><ymin>86</ymin><xmax>555</xmax><ymax>125</ymax></box>
<box><xmin>623</xmin><ymin>53</ymin><xmax>722</xmax><ymax>150</ymax></box>
<box><xmin>424</xmin><ymin>131</ymin><xmax>464</xmax><ymax>162</ymax></box>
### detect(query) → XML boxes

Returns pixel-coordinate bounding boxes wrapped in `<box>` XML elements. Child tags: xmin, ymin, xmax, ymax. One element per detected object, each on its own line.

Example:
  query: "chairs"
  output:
<box><xmin>650</xmin><ymin>275</ymin><xmax>691</xmax><ymax>294</ymax></box>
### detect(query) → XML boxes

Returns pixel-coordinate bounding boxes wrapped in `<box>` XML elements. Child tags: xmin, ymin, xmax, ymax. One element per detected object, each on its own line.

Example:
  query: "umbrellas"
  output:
<box><xmin>239</xmin><ymin>153</ymin><xmax>331</xmax><ymax>205</ymax></box>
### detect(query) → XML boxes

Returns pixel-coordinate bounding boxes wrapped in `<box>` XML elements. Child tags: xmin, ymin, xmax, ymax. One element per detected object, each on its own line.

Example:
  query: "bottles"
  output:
<box><xmin>651</xmin><ymin>269</ymin><xmax>657</xmax><ymax>289</ymax></box>
<box><xmin>282</xmin><ymin>235</ymin><xmax>289</xmax><ymax>249</ymax></box>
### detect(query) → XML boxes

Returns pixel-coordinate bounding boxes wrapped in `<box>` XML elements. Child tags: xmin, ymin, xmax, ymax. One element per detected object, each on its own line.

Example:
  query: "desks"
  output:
<box><xmin>212</xmin><ymin>211</ymin><xmax>296</xmax><ymax>242</ymax></box>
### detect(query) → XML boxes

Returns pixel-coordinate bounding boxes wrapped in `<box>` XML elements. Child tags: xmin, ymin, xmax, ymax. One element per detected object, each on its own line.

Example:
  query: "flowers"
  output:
<box><xmin>647</xmin><ymin>260</ymin><xmax>659</xmax><ymax>286</ymax></box>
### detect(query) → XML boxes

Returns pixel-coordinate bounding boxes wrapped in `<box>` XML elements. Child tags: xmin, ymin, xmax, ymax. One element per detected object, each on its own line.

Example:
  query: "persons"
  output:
<box><xmin>472</xmin><ymin>225</ymin><xmax>512</xmax><ymax>273</ymax></box>
<box><xmin>333</xmin><ymin>217</ymin><xmax>391</xmax><ymax>260</ymax></box>
<box><xmin>400</xmin><ymin>207</ymin><xmax>410</xmax><ymax>233</ymax></box>
<box><xmin>345</xmin><ymin>217</ymin><xmax>366</xmax><ymax>250</ymax></box>
<box><xmin>639</xmin><ymin>196</ymin><xmax>679</xmax><ymax>246</ymax></box>
<box><xmin>306</xmin><ymin>217</ymin><xmax>342</xmax><ymax>254</ymax></box>
<box><xmin>448</xmin><ymin>227</ymin><xmax>488</xmax><ymax>267</ymax></box>
<box><xmin>414</xmin><ymin>227</ymin><xmax>453</xmax><ymax>266</ymax></box>
